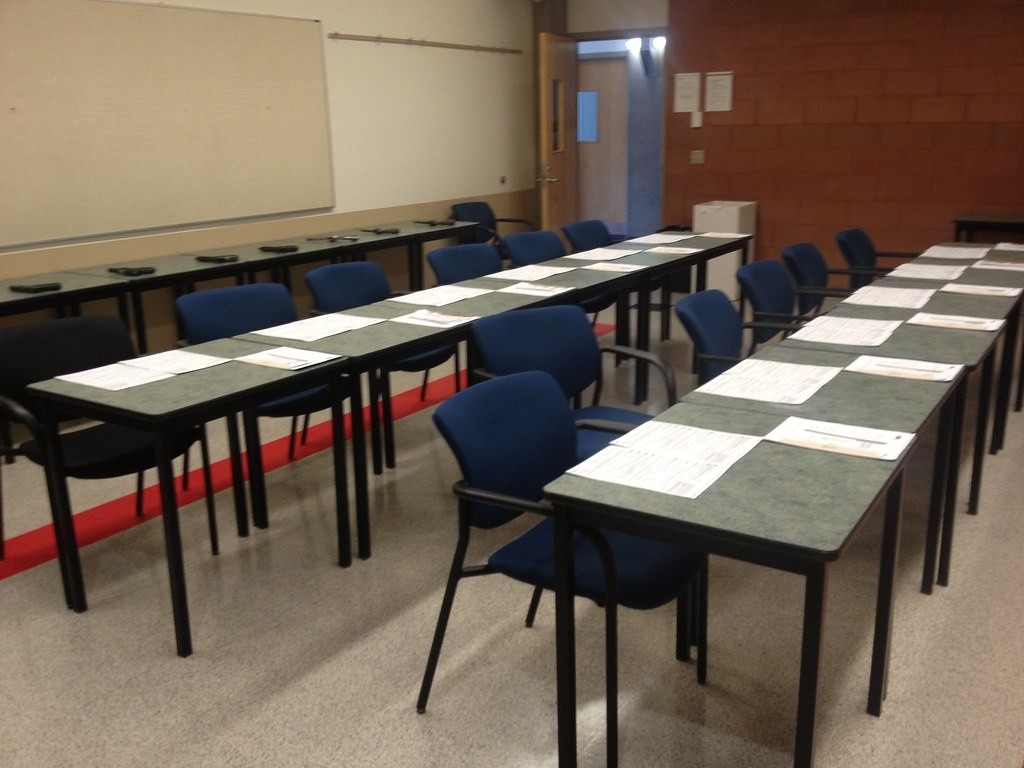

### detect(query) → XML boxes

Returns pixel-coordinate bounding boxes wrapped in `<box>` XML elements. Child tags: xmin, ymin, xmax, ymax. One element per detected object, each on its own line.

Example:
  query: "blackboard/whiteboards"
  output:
<box><xmin>0</xmin><ymin>0</ymin><xmax>338</xmax><ymax>254</ymax></box>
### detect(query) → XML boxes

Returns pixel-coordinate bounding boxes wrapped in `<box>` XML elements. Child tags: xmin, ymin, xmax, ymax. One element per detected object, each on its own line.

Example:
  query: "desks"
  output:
<box><xmin>952</xmin><ymin>213</ymin><xmax>1024</xmax><ymax>243</ymax></box>
<box><xmin>542</xmin><ymin>243</ymin><xmax>1024</xmax><ymax>768</ymax></box>
<box><xmin>0</xmin><ymin>219</ymin><xmax>481</xmax><ymax>563</ymax></box>
<box><xmin>24</xmin><ymin>228</ymin><xmax>759</xmax><ymax>658</ymax></box>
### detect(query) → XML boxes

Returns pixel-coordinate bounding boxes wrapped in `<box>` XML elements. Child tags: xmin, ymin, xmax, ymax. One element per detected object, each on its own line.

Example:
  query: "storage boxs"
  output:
<box><xmin>689</xmin><ymin>200</ymin><xmax>757</xmax><ymax>302</ymax></box>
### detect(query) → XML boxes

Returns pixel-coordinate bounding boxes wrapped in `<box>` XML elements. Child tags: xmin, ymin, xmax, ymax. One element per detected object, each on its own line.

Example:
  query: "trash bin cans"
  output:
<box><xmin>690</xmin><ymin>199</ymin><xmax>758</xmax><ymax>303</ymax></box>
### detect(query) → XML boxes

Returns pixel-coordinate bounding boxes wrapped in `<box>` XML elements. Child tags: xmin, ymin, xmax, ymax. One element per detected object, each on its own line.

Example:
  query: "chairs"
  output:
<box><xmin>782</xmin><ymin>243</ymin><xmax>891</xmax><ymax>335</ymax></box>
<box><xmin>834</xmin><ymin>229</ymin><xmax>922</xmax><ymax>292</ymax></box>
<box><xmin>426</xmin><ymin>244</ymin><xmax>503</xmax><ymax>286</ymax></box>
<box><xmin>449</xmin><ymin>202</ymin><xmax>536</xmax><ymax>270</ymax></box>
<box><xmin>469</xmin><ymin>304</ymin><xmax>679</xmax><ymax>462</ymax></box>
<box><xmin>167</xmin><ymin>283</ymin><xmax>354</xmax><ymax>528</ymax></box>
<box><xmin>416</xmin><ymin>371</ymin><xmax>704</xmax><ymax>767</ymax></box>
<box><xmin>302</xmin><ymin>260</ymin><xmax>461</xmax><ymax>469</ymax></box>
<box><xmin>0</xmin><ymin>316</ymin><xmax>220</xmax><ymax>614</ymax></box>
<box><xmin>500</xmin><ymin>231</ymin><xmax>616</xmax><ymax>313</ymax></box>
<box><xmin>675</xmin><ymin>289</ymin><xmax>804</xmax><ymax>389</ymax></box>
<box><xmin>735</xmin><ymin>259</ymin><xmax>853</xmax><ymax>357</ymax></box>
<box><xmin>562</xmin><ymin>220</ymin><xmax>663</xmax><ymax>294</ymax></box>
